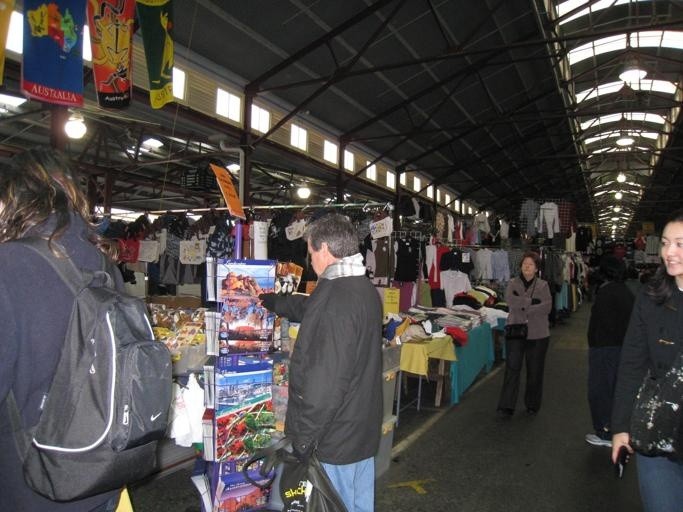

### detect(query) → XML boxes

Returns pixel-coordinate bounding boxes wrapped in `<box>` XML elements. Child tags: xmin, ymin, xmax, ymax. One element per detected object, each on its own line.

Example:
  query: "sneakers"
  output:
<box><xmin>585</xmin><ymin>433</ymin><xmax>613</xmax><ymax>447</ymax></box>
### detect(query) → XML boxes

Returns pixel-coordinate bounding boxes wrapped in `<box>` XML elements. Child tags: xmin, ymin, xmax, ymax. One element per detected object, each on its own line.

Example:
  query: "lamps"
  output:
<box><xmin>297</xmin><ymin>178</ymin><xmax>312</xmax><ymax>198</ymax></box>
<box><xmin>64</xmin><ymin>107</ymin><xmax>87</xmax><ymax>139</ymax></box>
<box><xmin>616</xmin><ymin>131</ymin><xmax>634</xmax><ymax>147</ymax></box>
<box><xmin>617</xmin><ymin>170</ymin><xmax>626</xmax><ymax>183</ymax></box>
<box><xmin>620</xmin><ymin>56</ymin><xmax>648</xmax><ymax>84</ymax></box>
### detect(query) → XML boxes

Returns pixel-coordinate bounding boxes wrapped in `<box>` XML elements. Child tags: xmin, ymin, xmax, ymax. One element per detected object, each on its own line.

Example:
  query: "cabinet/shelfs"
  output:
<box><xmin>374</xmin><ymin>344</ymin><xmax>402</xmax><ymax>481</ymax></box>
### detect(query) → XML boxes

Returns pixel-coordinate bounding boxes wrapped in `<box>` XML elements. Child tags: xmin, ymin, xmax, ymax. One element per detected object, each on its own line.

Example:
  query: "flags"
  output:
<box><xmin>88</xmin><ymin>0</ymin><xmax>137</xmax><ymax>109</ymax></box>
<box><xmin>138</xmin><ymin>1</ymin><xmax>175</xmax><ymax>109</ymax></box>
<box><xmin>22</xmin><ymin>1</ymin><xmax>89</xmax><ymax>110</ymax></box>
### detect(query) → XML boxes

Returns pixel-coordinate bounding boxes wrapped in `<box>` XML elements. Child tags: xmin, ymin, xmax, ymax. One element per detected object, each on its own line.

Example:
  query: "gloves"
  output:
<box><xmin>512</xmin><ymin>288</ymin><xmax>519</xmax><ymax>300</ymax></box>
<box><xmin>531</xmin><ymin>297</ymin><xmax>542</xmax><ymax>306</ymax></box>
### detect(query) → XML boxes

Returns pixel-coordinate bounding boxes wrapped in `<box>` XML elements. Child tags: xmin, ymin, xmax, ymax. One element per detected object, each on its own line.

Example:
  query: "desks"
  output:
<box><xmin>392</xmin><ymin>334</ymin><xmax>454</xmax><ymax>425</ymax></box>
<box><xmin>413</xmin><ymin>305</ymin><xmax>508</xmax><ymax>405</ymax></box>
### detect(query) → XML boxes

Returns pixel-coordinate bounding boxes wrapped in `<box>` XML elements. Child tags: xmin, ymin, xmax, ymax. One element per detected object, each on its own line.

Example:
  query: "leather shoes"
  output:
<box><xmin>496</xmin><ymin>406</ymin><xmax>515</xmax><ymax>417</ymax></box>
<box><xmin>522</xmin><ymin>406</ymin><xmax>537</xmax><ymax>417</ymax></box>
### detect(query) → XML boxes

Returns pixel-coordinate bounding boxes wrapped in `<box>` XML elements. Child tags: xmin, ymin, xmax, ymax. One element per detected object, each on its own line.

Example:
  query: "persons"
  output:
<box><xmin>584</xmin><ymin>253</ymin><xmax>636</xmax><ymax>448</ymax></box>
<box><xmin>497</xmin><ymin>255</ymin><xmax>552</xmax><ymax>417</ymax></box>
<box><xmin>609</xmin><ymin>212</ymin><xmax>683</xmax><ymax>512</ymax></box>
<box><xmin>0</xmin><ymin>149</ymin><xmax>122</xmax><ymax>512</ymax></box>
<box><xmin>259</xmin><ymin>214</ymin><xmax>383</xmax><ymax>512</ymax></box>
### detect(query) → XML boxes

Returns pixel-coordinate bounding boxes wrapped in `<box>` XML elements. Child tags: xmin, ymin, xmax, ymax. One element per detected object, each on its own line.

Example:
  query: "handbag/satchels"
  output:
<box><xmin>243</xmin><ymin>439</ymin><xmax>350</xmax><ymax>512</ymax></box>
<box><xmin>628</xmin><ymin>355</ymin><xmax>683</xmax><ymax>457</ymax></box>
<box><xmin>504</xmin><ymin>276</ymin><xmax>537</xmax><ymax>340</ymax></box>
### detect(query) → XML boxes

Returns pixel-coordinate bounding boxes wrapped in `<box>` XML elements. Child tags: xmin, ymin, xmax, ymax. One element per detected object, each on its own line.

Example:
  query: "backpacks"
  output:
<box><xmin>1</xmin><ymin>237</ymin><xmax>173</xmax><ymax>503</ymax></box>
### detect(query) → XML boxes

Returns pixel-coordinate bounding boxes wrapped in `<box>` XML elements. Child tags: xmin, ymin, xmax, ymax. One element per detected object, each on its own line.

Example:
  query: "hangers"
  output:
<box><xmin>301</xmin><ymin>203</ymin><xmax>310</xmax><ymax>212</ymax></box>
<box><xmin>182</xmin><ymin>208</ymin><xmax>200</xmax><ymax>217</ymax></box>
<box><xmin>341</xmin><ymin>202</ymin><xmax>346</xmax><ymax>212</ymax></box>
<box><xmin>381</xmin><ymin>200</ymin><xmax>391</xmax><ymax>212</ymax></box>
<box><xmin>361</xmin><ymin>201</ymin><xmax>371</xmax><ymax>213</ymax></box>
